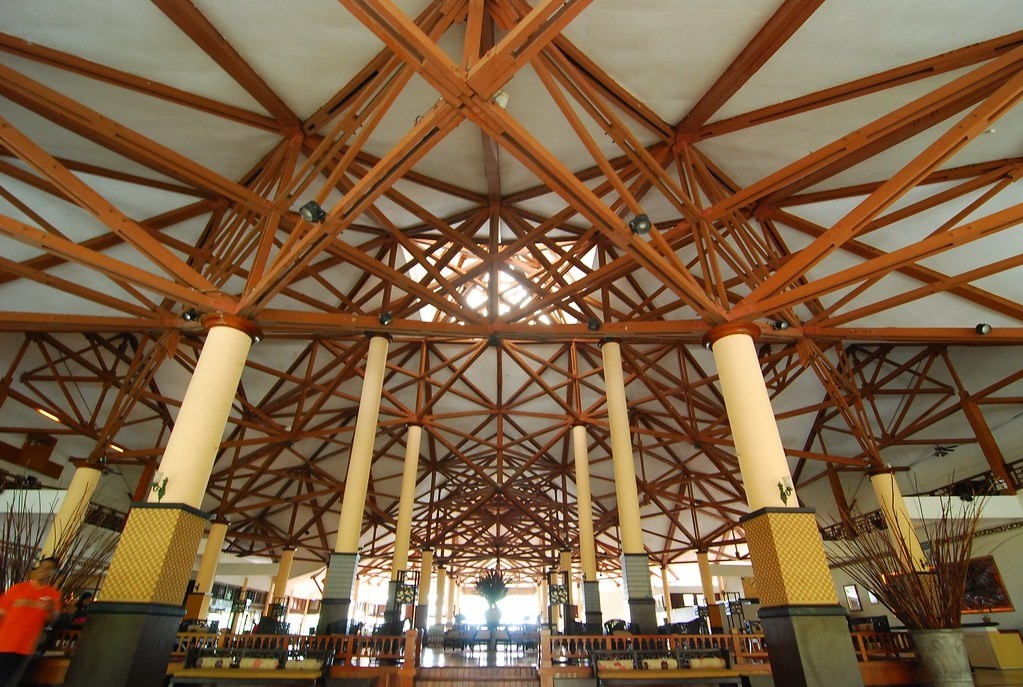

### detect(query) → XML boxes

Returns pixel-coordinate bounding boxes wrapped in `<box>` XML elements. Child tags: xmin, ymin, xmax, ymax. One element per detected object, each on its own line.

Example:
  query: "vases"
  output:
<box><xmin>909</xmin><ymin>628</ymin><xmax>977</xmax><ymax>687</ymax></box>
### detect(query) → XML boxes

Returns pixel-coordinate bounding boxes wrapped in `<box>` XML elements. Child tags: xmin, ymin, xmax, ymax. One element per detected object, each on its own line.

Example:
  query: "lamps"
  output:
<box><xmin>183</xmin><ymin>309</ymin><xmax>199</xmax><ymax>323</ymax></box>
<box><xmin>628</xmin><ymin>215</ymin><xmax>652</xmax><ymax>234</ymax></box>
<box><xmin>379</xmin><ymin>312</ymin><xmax>394</xmax><ymax>326</ymax></box>
<box><xmin>586</xmin><ymin>319</ymin><xmax>600</xmax><ymax>330</ymax></box>
<box><xmin>775</xmin><ymin>320</ymin><xmax>789</xmax><ymax>330</ymax></box>
<box><xmin>976</xmin><ymin>323</ymin><xmax>991</xmax><ymax>334</ymax></box>
<box><xmin>300</xmin><ymin>201</ymin><xmax>327</xmax><ymax>223</ymax></box>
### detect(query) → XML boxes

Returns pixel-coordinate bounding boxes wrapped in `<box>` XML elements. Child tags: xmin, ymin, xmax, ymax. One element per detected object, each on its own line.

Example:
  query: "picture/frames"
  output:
<box><xmin>843</xmin><ymin>584</ymin><xmax>864</xmax><ymax>612</ymax></box>
<box><xmin>929</xmin><ymin>553</ymin><xmax>1019</xmax><ymax>614</ymax></box>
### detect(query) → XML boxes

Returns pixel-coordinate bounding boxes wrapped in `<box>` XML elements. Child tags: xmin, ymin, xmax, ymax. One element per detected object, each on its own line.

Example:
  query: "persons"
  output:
<box><xmin>0</xmin><ymin>556</ymin><xmax>62</xmax><ymax>687</ymax></box>
<box><xmin>72</xmin><ymin>592</ymin><xmax>92</xmax><ymax>629</ymax></box>
